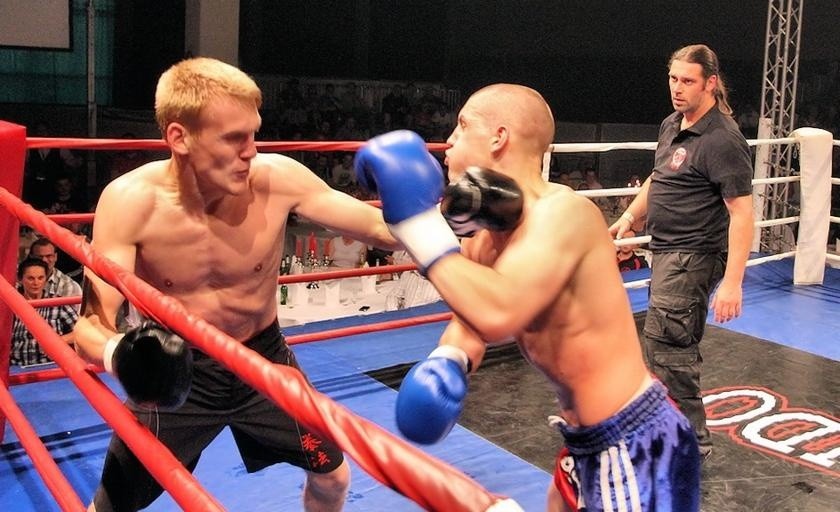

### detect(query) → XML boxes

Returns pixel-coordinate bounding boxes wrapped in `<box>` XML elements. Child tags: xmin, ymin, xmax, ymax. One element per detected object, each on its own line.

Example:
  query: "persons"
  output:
<box><xmin>354</xmin><ymin>84</ymin><xmax>704</xmax><ymax>510</ymax></box>
<box><xmin>254</xmin><ymin>79</ymin><xmax>476</xmax><ymax>186</ymax></box>
<box><xmin>732</xmin><ymin>102</ymin><xmax>840</xmax><ymax>253</ymax></box>
<box><xmin>557</xmin><ymin>165</ymin><xmax>654</xmax><ymax>272</ymax></box>
<box><xmin>605</xmin><ymin>43</ymin><xmax>757</xmax><ymax>462</ymax></box>
<box><xmin>74</xmin><ymin>56</ymin><xmax>524</xmax><ymax>510</ymax></box>
<box><xmin>329</xmin><ymin>233</ymin><xmax>413</xmax><ymax>265</ymax></box>
<box><xmin>11</xmin><ymin>126</ymin><xmax>173</xmax><ymax>366</ymax></box>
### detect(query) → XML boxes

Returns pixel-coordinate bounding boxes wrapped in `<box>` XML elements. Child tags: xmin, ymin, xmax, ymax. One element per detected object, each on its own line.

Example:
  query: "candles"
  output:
<box><xmin>296</xmin><ymin>231</ymin><xmax>329</xmax><ymax>257</ymax></box>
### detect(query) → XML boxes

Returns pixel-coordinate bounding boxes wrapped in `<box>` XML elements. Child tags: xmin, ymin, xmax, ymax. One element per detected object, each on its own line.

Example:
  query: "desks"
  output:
<box><xmin>277</xmin><ymin>267</ymin><xmax>401</xmax><ymax>328</ymax></box>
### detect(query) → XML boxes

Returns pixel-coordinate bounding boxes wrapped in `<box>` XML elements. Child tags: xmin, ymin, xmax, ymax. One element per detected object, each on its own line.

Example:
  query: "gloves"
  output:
<box><xmin>357</xmin><ymin>129</ymin><xmax>460</xmax><ymax>273</ymax></box>
<box><xmin>394</xmin><ymin>345</ymin><xmax>472</xmax><ymax>443</ymax></box>
<box><xmin>441</xmin><ymin>164</ymin><xmax>523</xmax><ymax>241</ymax></box>
<box><xmin>101</xmin><ymin>321</ymin><xmax>193</xmax><ymax>414</ymax></box>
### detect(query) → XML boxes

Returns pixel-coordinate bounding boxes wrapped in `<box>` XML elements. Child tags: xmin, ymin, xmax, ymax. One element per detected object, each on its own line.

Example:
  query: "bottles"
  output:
<box><xmin>280</xmin><ymin>253</ymin><xmax>298</xmax><ymax>305</ymax></box>
